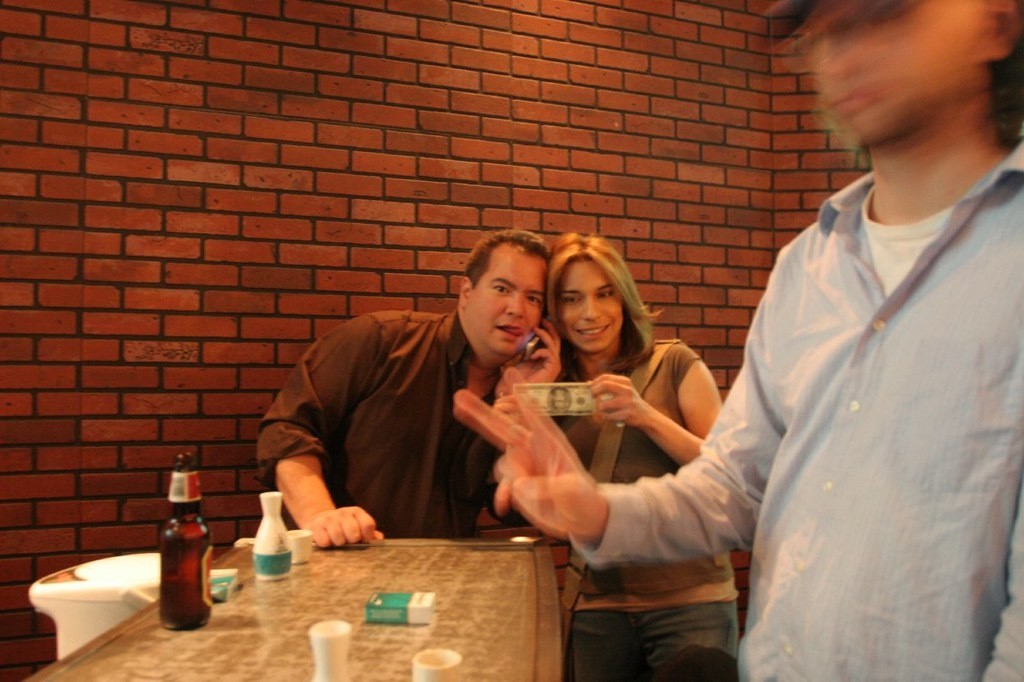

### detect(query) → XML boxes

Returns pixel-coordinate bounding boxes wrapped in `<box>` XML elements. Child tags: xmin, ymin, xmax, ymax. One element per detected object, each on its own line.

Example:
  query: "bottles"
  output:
<box><xmin>251</xmin><ymin>491</ymin><xmax>292</xmax><ymax>581</ymax></box>
<box><xmin>160</xmin><ymin>451</ymin><xmax>212</xmax><ymax>629</ymax></box>
<box><xmin>307</xmin><ymin>619</ymin><xmax>350</xmax><ymax>682</ymax></box>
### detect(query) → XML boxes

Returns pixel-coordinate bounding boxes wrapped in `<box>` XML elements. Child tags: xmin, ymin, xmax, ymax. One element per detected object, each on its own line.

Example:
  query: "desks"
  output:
<box><xmin>22</xmin><ymin>536</ymin><xmax>563</xmax><ymax>681</ymax></box>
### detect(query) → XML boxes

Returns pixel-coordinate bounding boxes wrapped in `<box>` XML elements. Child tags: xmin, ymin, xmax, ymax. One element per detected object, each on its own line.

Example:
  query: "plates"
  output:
<box><xmin>75</xmin><ymin>553</ymin><xmax>160</xmax><ymax>590</ymax></box>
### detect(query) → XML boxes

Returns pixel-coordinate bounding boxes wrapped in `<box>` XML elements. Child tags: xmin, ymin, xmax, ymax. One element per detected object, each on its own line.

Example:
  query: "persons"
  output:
<box><xmin>452</xmin><ymin>0</ymin><xmax>1024</xmax><ymax>682</ymax></box>
<box><xmin>260</xmin><ymin>232</ymin><xmax>738</xmax><ymax>682</ymax></box>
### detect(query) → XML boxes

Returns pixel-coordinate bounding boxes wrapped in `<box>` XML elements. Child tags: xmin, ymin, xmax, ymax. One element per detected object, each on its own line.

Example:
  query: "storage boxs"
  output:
<box><xmin>365</xmin><ymin>591</ymin><xmax>438</xmax><ymax>627</ymax></box>
<box><xmin>209</xmin><ymin>568</ymin><xmax>239</xmax><ymax>602</ymax></box>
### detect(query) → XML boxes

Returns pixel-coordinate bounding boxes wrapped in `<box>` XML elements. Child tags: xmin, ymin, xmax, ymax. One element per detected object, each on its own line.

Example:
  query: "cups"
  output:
<box><xmin>412</xmin><ymin>648</ymin><xmax>463</xmax><ymax>682</ymax></box>
<box><xmin>288</xmin><ymin>529</ymin><xmax>312</xmax><ymax>564</ymax></box>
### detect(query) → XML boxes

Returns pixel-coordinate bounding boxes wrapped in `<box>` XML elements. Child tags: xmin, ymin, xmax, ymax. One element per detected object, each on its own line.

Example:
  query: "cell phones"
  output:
<box><xmin>522</xmin><ymin>315</ymin><xmax>551</xmax><ymax>361</ymax></box>
